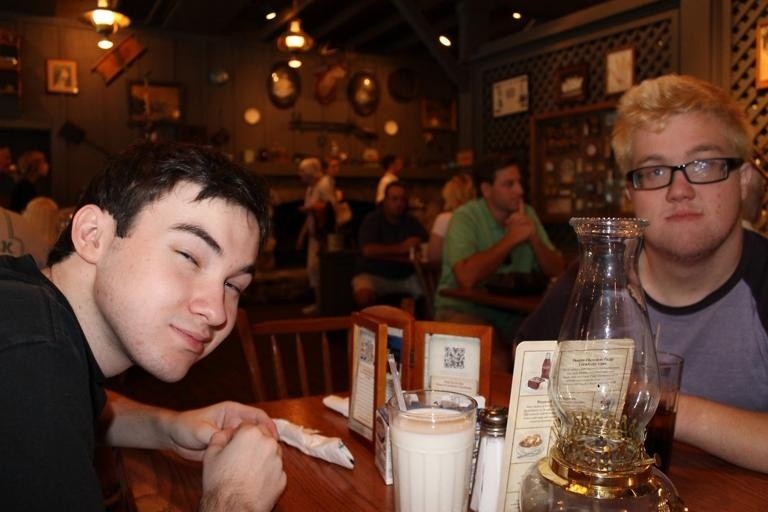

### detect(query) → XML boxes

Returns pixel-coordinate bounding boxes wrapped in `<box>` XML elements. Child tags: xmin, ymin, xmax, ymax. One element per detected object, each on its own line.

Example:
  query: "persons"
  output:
<box><xmin>222</xmin><ymin>153</ymin><xmax>476</xmax><ymax>312</ymax></box>
<box><xmin>510</xmin><ymin>74</ymin><xmax>768</xmax><ymax>475</ymax></box>
<box><xmin>0</xmin><ymin>138</ymin><xmax>289</xmax><ymax>511</ymax></box>
<box><xmin>437</xmin><ymin>153</ymin><xmax>566</xmax><ymax>371</ymax></box>
<box><xmin>1</xmin><ymin>145</ymin><xmax>43</xmax><ymax>216</ymax></box>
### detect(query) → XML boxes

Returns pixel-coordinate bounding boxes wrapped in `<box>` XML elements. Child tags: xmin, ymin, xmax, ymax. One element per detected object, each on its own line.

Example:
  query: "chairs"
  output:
<box><xmin>411</xmin><ymin>257</ymin><xmax>443</xmax><ymax>324</ymax></box>
<box><xmin>231</xmin><ymin>297</ymin><xmax>414</xmax><ymax>402</ymax></box>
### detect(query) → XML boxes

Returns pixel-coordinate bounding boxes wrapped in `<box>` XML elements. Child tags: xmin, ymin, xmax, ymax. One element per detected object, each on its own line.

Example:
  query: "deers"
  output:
<box><xmin>313</xmin><ymin>42</ymin><xmax>359</xmax><ymax>106</ymax></box>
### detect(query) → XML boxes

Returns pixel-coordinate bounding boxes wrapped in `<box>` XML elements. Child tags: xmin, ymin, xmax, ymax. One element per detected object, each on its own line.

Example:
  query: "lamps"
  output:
<box><xmin>276</xmin><ymin>1</ymin><xmax>314</xmax><ymax>69</ymax></box>
<box><xmin>85</xmin><ymin>0</ymin><xmax>131</xmax><ymax>49</ymax></box>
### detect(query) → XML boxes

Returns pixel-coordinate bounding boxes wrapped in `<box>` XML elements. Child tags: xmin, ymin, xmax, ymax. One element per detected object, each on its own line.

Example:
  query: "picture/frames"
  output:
<box><xmin>551</xmin><ymin>61</ymin><xmax>591</xmax><ymax>104</ymax></box>
<box><xmin>125</xmin><ymin>77</ymin><xmax>189</xmax><ymax>129</ymax></box>
<box><xmin>603</xmin><ymin>43</ymin><xmax>637</xmax><ymax>99</ymax></box>
<box><xmin>47</xmin><ymin>59</ymin><xmax>78</xmax><ymax>94</ymax></box>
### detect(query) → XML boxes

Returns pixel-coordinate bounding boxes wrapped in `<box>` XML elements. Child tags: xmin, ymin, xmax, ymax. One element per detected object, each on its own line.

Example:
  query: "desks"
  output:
<box><xmin>119</xmin><ymin>367</ymin><xmax>767</xmax><ymax>510</ymax></box>
<box><xmin>436</xmin><ymin>270</ymin><xmax>536</xmax><ymax>312</ymax></box>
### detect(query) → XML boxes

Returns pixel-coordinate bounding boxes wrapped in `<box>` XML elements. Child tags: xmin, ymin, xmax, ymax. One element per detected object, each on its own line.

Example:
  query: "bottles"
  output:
<box><xmin>540</xmin><ymin>353</ymin><xmax>551</xmax><ymax>379</ymax></box>
<box><xmin>469</xmin><ymin>408</ymin><xmax>506</xmax><ymax>512</ymax></box>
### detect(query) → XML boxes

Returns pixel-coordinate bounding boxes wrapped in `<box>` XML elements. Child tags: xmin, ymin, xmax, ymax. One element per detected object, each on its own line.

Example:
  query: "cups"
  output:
<box><xmin>387</xmin><ymin>386</ymin><xmax>479</xmax><ymax>512</ymax></box>
<box><xmin>630</xmin><ymin>349</ymin><xmax>684</xmax><ymax>464</ymax></box>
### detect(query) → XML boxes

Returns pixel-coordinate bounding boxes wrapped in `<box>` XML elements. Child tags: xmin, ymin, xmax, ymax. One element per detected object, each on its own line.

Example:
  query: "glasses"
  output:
<box><xmin>624</xmin><ymin>157</ymin><xmax>746</xmax><ymax>191</ymax></box>
